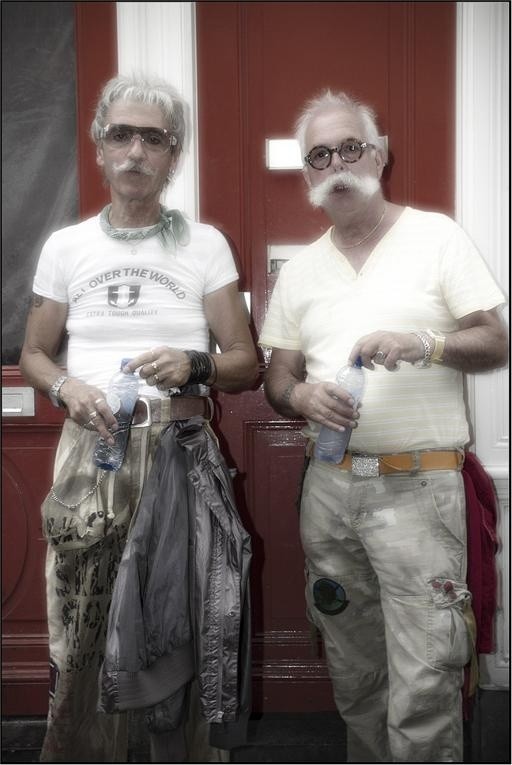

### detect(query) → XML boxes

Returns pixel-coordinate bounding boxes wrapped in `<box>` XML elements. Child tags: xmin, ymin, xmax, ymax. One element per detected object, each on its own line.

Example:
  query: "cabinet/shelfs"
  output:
<box><xmin>91</xmin><ymin>355</ymin><xmax>141</xmax><ymax>472</ymax></box>
<box><xmin>312</xmin><ymin>353</ymin><xmax>365</xmax><ymax>465</ymax></box>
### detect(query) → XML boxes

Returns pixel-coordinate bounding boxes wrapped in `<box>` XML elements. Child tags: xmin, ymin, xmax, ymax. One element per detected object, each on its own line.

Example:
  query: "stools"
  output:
<box><xmin>61</xmin><ymin>393</ymin><xmax>210</xmax><ymax>427</ymax></box>
<box><xmin>303</xmin><ymin>437</ymin><xmax>462</xmax><ymax>477</ymax></box>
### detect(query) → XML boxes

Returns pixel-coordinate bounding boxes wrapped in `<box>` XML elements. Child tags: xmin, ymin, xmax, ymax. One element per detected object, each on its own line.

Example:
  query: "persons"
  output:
<box><xmin>259</xmin><ymin>85</ymin><xmax>509</xmax><ymax>764</ymax></box>
<box><xmin>15</xmin><ymin>72</ymin><xmax>260</xmax><ymax>764</ymax></box>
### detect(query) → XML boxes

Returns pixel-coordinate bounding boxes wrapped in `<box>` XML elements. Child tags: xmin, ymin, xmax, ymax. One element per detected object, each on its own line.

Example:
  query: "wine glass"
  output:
<box><xmin>92</xmin><ymin>398</ymin><xmax>106</xmax><ymax>410</ymax></box>
<box><xmin>149</xmin><ymin>347</ymin><xmax>160</xmax><ymax>360</ymax></box>
<box><xmin>153</xmin><ymin>373</ymin><xmax>160</xmax><ymax>384</ymax></box>
<box><xmin>79</xmin><ymin>421</ymin><xmax>95</xmax><ymax>431</ymax></box>
<box><xmin>86</xmin><ymin>411</ymin><xmax>100</xmax><ymax>421</ymax></box>
<box><xmin>374</xmin><ymin>348</ymin><xmax>385</xmax><ymax>362</ymax></box>
<box><xmin>149</xmin><ymin>359</ymin><xmax>160</xmax><ymax>371</ymax></box>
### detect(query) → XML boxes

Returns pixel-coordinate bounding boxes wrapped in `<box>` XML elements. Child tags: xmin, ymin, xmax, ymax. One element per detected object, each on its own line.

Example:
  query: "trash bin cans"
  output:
<box><xmin>47</xmin><ymin>375</ymin><xmax>70</xmax><ymax>411</ymax></box>
<box><xmin>417</xmin><ymin>327</ymin><xmax>446</xmax><ymax>364</ymax></box>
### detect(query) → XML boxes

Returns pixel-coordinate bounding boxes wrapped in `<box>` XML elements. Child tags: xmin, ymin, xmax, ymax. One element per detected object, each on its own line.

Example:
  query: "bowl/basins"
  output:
<box><xmin>328</xmin><ymin>197</ymin><xmax>388</xmax><ymax>249</ymax></box>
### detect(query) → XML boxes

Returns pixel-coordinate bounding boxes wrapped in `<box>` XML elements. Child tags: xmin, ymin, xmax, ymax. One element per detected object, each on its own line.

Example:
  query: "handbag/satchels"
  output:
<box><xmin>409</xmin><ymin>327</ymin><xmax>431</xmax><ymax>372</ymax></box>
<box><xmin>184</xmin><ymin>349</ymin><xmax>218</xmax><ymax>388</ymax></box>
<box><xmin>280</xmin><ymin>378</ymin><xmax>305</xmax><ymax>419</ymax></box>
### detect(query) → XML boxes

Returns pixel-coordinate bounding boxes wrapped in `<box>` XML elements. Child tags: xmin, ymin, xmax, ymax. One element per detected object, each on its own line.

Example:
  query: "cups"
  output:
<box><xmin>99</xmin><ymin>122</ymin><xmax>179</xmax><ymax>154</ymax></box>
<box><xmin>303</xmin><ymin>136</ymin><xmax>375</xmax><ymax>171</ymax></box>
